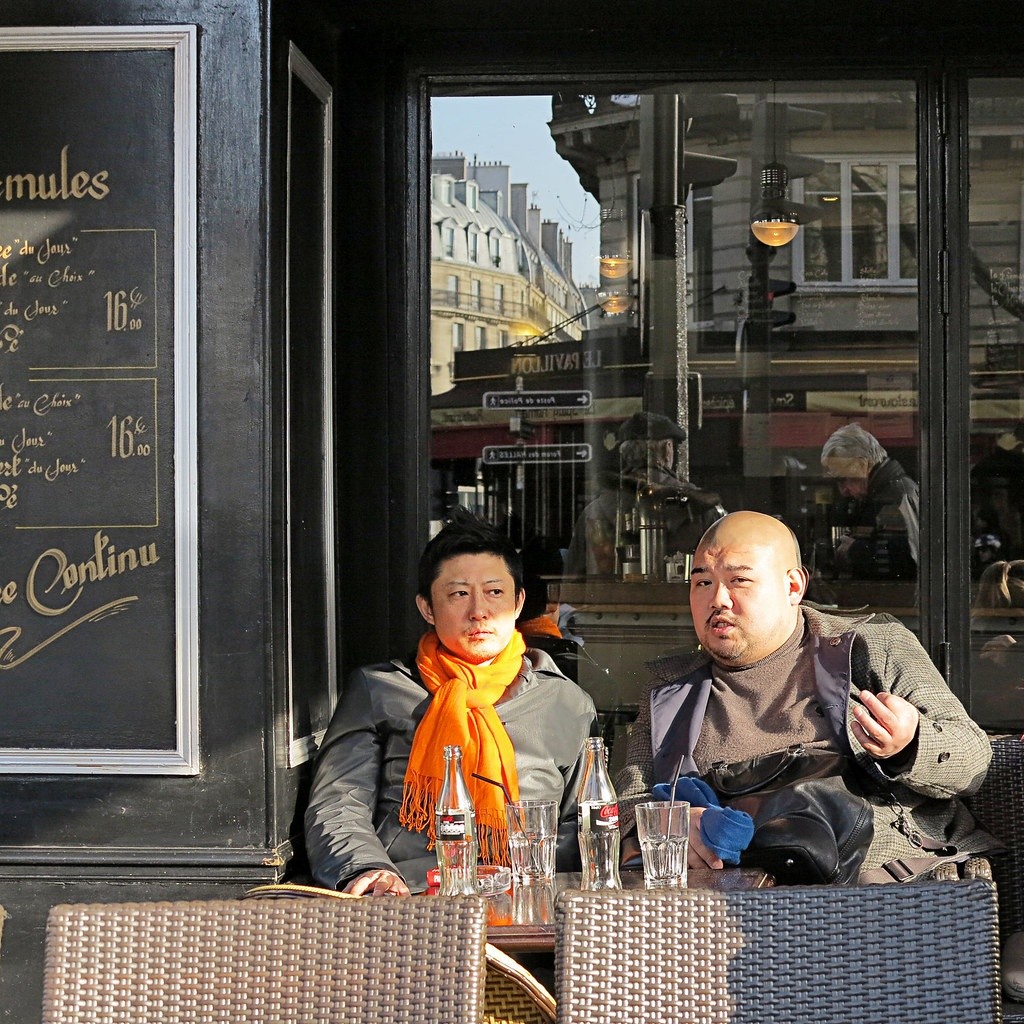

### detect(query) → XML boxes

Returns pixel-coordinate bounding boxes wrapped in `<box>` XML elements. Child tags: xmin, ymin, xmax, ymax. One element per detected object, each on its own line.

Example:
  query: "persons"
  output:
<box><xmin>612</xmin><ymin>512</ymin><xmax>995</xmax><ymax>886</ymax></box>
<box><xmin>562</xmin><ymin>411</ymin><xmax>725</xmax><ymax>581</ymax></box>
<box><xmin>300</xmin><ymin>521</ymin><xmax>604</xmax><ymax>894</ymax></box>
<box><xmin>820</xmin><ymin>423</ymin><xmax>923</xmax><ymax>584</ymax></box>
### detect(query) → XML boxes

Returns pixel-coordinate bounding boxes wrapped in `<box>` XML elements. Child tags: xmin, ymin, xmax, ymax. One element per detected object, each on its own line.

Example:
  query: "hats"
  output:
<box><xmin>617</xmin><ymin>412</ymin><xmax>686</xmax><ymax>440</ymax></box>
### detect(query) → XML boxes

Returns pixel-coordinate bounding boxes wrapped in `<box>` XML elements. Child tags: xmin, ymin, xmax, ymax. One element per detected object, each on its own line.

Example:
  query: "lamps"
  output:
<box><xmin>589</xmin><ymin>205</ymin><xmax>635</xmax><ymax>279</ymax></box>
<box><xmin>748</xmin><ymin>82</ymin><xmax>800</xmax><ymax>246</ymax></box>
<box><xmin>594</xmin><ymin>278</ymin><xmax>633</xmax><ymax>314</ymax></box>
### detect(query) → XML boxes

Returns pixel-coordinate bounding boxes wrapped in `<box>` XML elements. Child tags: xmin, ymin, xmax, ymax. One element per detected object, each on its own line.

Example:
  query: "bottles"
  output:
<box><xmin>576</xmin><ymin>739</ymin><xmax>624</xmax><ymax>891</ymax></box>
<box><xmin>434</xmin><ymin>745</ymin><xmax>478</xmax><ymax>897</ymax></box>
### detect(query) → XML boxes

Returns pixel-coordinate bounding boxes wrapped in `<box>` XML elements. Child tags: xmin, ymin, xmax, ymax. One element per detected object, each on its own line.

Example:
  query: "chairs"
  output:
<box><xmin>239</xmin><ymin>884</ymin><xmax>556</xmax><ymax>1024</ymax></box>
<box><xmin>962</xmin><ymin>733</ymin><xmax>1024</xmax><ymax>1024</ymax></box>
<box><xmin>41</xmin><ymin>892</ymin><xmax>490</xmax><ymax>1024</ymax></box>
<box><xmin>554</xmin><ymin>878</ymin><xmax>1004</xmax><ymax>1024</ymax></box>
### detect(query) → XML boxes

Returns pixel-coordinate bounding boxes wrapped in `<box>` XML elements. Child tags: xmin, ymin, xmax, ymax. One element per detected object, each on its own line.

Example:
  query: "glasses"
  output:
<box><xmin>827</xmin><ymin>459</ymin><xmax>855</xmax><ymax>479</ymax></box>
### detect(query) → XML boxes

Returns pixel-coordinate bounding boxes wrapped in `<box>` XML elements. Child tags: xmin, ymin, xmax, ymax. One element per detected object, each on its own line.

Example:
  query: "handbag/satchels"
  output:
<box><xmin>697</xmin><ymin>744</ymin><xmax>875</xmax><ymax>886</ymax></box>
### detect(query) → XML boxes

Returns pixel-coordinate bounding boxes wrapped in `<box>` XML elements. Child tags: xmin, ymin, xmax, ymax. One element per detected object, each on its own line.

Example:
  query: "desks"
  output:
<box><xmin>487</xmin><ymin>869</ymin><xmax>774</xmax><ymax>965</ymax></box>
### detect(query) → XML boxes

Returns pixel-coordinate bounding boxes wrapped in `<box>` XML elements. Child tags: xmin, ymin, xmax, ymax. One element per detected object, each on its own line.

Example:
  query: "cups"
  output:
<box><xmin>636</xmin><ymin>800</ymin><xmax>691</xmax><ymax>891</ymax></box>
<box><xmin>504</xmin><ymin>800</ymin><xmax>560</xmax><ymax>880</ymax></box>
